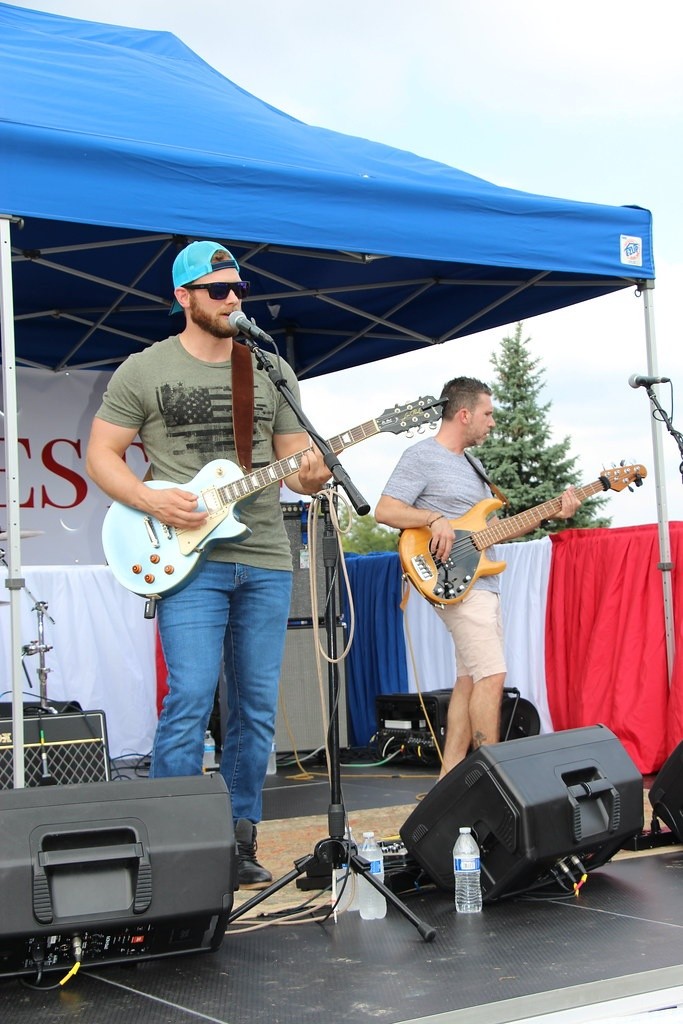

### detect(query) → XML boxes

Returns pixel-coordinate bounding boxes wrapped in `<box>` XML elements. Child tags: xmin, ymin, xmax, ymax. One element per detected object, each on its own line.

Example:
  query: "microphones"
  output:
<box><xmin>629</xmin><ymin>374</ymin><xmax>671</xmax><ymax>388</ymax></box>
<box><xmin>38</xmin><ymin>718</ymin><xmax>57</xmax><ymax>786</ymax></box>
<box><xmin>228</xmin><ymin>311</ymin><xmax>275</xmax><ymax>345</ymax></box>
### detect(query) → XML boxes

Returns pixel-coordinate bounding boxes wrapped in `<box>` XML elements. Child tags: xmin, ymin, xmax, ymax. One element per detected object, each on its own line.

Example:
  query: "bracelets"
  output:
<box><xmin>429</xmin><ymin>516</ymin><xmax>444</xmax><ymax>528</ymax></box>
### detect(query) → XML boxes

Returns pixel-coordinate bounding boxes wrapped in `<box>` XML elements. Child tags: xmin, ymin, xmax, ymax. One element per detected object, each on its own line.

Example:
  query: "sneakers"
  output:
<box><xmin>231</xmin><ymin>818</ymin><xmax>272</xmax><ymax>890</ymax></box>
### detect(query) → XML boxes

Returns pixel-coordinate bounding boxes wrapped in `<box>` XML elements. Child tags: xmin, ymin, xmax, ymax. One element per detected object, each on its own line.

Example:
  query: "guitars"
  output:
<box><xmin>100</xmin><ymin>394</ymin><xmax>451</xmax><ymax>602</ymax></box>
<box><xmin>397</xmin><ymin>458</ymin><xmax>648</xmax><ymax>608</ymax></box>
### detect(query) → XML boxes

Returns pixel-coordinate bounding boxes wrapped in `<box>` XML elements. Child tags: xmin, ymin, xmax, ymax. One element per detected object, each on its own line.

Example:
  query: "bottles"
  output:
<box><xmin>357</xmin><ymin>832</ymin><xmax>387</xmax><ymax>920</ymax></box>
<box><xmin>202</xmin><ymin>731</ymin><xmax>215</xmax><ymax>767</ymax></box>
<box><xmin>453</xmin><ymin>827</ymin><xmax>483</xmax><ymax>913</ymax></box>
<box><xmin>336</xmin><ymin>827</ymin><xmax>359</xmax><ymax>911</ymax></box>
<box><xmin>266</xmin><ymin>737</ymin><xmax>276</xmax><ymax>775</ymax></box>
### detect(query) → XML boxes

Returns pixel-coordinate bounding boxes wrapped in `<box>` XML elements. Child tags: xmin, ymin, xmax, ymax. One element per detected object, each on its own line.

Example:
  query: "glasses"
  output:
<box><xmin>184</xmin><ymin>281</ymin><xmax>250</xmax><ymax>301</ymax></box>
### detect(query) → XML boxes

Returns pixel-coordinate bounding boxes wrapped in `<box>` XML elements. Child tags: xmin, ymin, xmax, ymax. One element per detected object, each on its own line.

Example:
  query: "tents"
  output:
<box><xmin>0</xmin><ymin>2</ymin><xmax>675</xmax><ymax>791</ymax></box>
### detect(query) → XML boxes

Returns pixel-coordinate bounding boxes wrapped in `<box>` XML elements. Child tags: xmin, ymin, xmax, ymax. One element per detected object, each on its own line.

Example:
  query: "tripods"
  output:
<box><xmin>228</xmin><ymin>335</ymin><xmax>438</xmax><ymax>941</ymax></box>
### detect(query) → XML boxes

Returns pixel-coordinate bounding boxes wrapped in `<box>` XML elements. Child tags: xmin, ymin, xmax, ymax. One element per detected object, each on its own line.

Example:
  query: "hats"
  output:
<box><xmin>169</xmin><ymin>240</ymin><xmax>238</xmax><ymax>315</ymax></box>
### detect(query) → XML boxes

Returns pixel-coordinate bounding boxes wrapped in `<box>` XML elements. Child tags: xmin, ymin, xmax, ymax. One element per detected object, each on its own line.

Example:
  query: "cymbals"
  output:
<box><xmin>0</xmin><ymin>529</ymin><xmax>46</xmax><ymax>541</ymax></box>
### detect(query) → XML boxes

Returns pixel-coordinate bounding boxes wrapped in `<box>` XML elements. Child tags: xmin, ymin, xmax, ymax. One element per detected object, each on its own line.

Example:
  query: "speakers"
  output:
<box><xmin>275</xmin><ymin>501</ymin><xmax>345</xmax><ymax>622</ymax></box>
<box><xmin>399</xmin><ymin>723</ymin><xmax>645</xmax><ymax>901</ymax></box>
<box><xmin>373</xmin><ymin>691</ymin><xmax>512</xmax><ymax>767</ymax></box>
<box><xmin>0</xmin><ymin>709</ymin><xmax>112</xmax><ymax>791</ymax></box>
<box><xmin>219</xmin><ymin>621</ymin><xmax>350</xmax><ymax>755</ymax></box>
<box><xmin>0</xmin><ymin>771</ymin><xmax>239</xmax><ymax>984</ymax></box>
<box><xmin>648</xmin><ymin>740</ymin><xmax>683</xmax><ymax>845</ymax></box>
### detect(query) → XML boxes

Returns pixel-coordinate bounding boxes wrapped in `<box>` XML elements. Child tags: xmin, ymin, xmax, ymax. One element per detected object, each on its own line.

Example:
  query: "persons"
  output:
<box><xmin>86</xmin><ymin>241</ymin><xmax>334</xmax><ymax>889</ymax></box>
<box><xmin>374</xmin><ymin>378</ymin><xmax>581</xmax><ymax>783</ymax></box>
